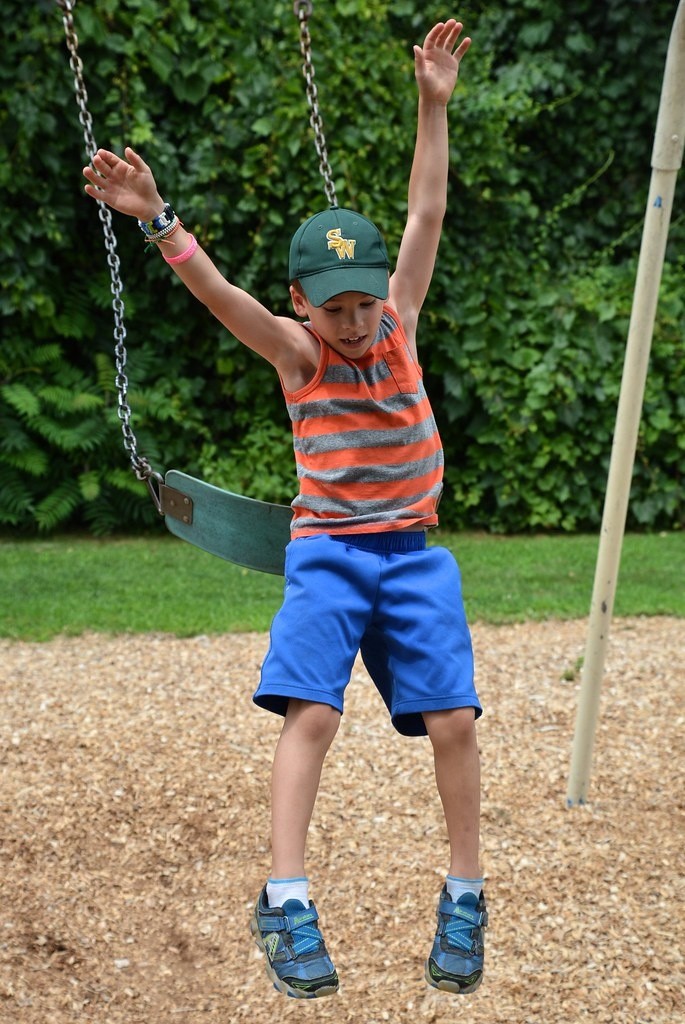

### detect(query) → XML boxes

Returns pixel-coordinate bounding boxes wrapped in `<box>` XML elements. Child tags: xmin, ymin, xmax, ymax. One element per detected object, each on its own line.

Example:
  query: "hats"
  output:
<box><xmin>288</xmin><ymin>205</ymin><xmax>390</xmax><ymax>307</ymax></box>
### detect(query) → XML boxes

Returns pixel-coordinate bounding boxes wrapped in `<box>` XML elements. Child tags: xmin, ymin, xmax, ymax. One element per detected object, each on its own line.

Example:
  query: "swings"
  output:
<box><xmin>54</xmin><ymin>0</ymin><xmax>340</xmax><ymax>578</ymax></box>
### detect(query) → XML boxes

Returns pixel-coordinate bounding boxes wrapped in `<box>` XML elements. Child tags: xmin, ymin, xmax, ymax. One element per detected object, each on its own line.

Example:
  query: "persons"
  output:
<box><xmin>79</xmin><ymin>15</ymin><xmax>490</xmax><ymax>1002</ymax></box>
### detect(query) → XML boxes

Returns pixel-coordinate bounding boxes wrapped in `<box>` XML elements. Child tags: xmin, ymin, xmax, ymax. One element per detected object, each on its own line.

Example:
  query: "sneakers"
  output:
<box><xmin>251</xmin><ymin>882</ymin><xmax>340</xmax><ymax>998</ymax></box>
<box><xmin>425</xmin><ymin>882</ymin><xmax>489</xmax><ymax>994</ymax></box>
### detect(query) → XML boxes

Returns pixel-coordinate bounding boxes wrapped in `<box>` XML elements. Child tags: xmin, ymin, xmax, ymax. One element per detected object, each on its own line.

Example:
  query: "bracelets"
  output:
<box><xmin>161</xmin><ymin>233</ymin><xmax>199</xmax><ymax>266</ymax></box>
<box><xmin>137</xmin><ymin>202</ymin><xmax>175</xmax><ymax>237</ymax></box>
<box><xmin>146</xmin><ymin>214</ymin><xmax>179</xmax><ymax>239</ymax></box>
<box><xmin>142</xmin><ymin>219</ymin><xmax>185</xmax><ymax>253</ymax></box>
